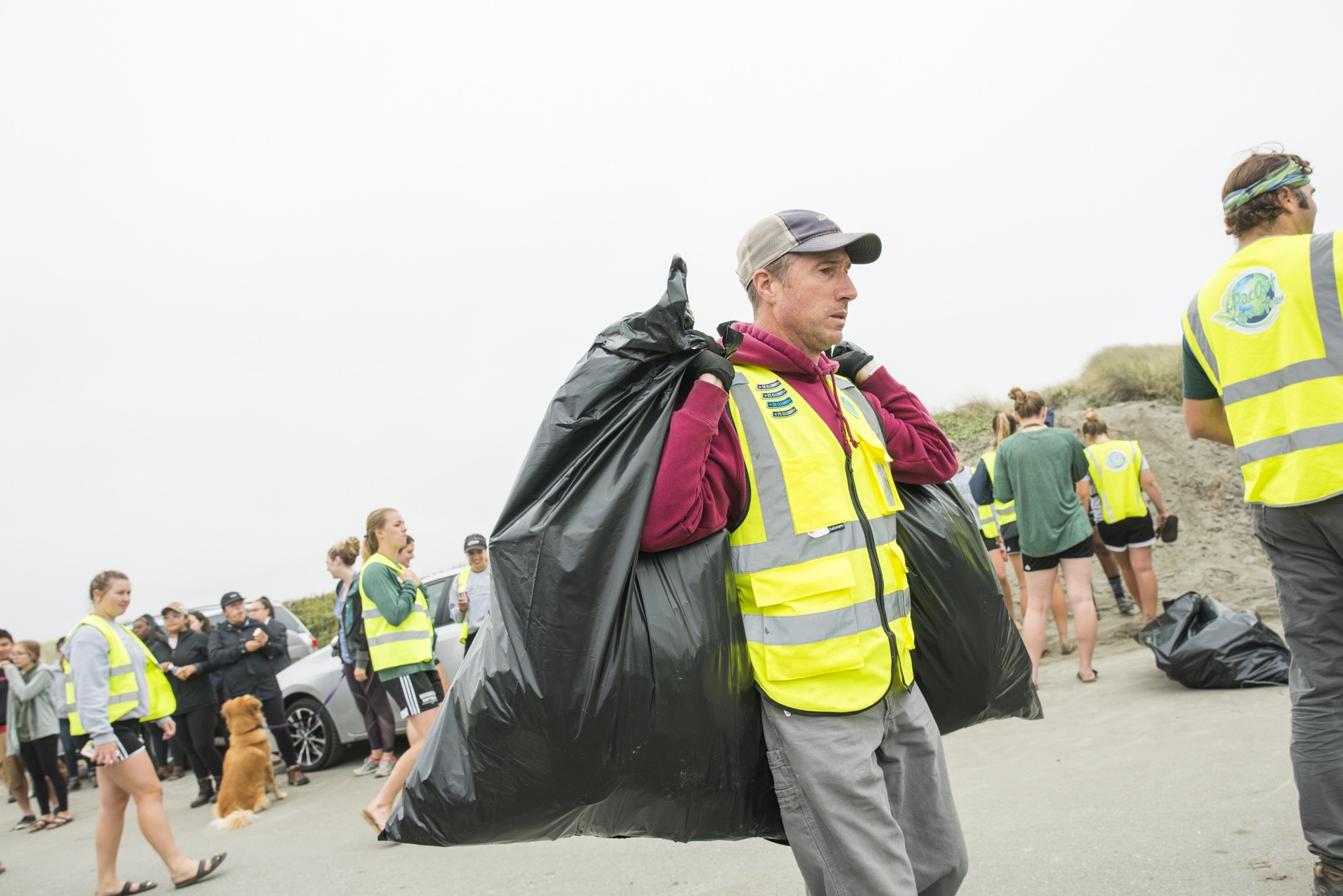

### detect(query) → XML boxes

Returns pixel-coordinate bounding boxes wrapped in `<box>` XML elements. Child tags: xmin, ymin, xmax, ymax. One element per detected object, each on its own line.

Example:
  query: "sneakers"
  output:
<box><xmin>376</xmin><ymin>755</ymin><xmax>397</xmax><ymax>776</ymax></box>
<box><xmin>14</xmin><ymin>814</ymin><xmax>36</xmax><ymax>829</ymax></box>
<box><xmin>353</xmin><ymin>755</ymin><xmax>381</xmax><ymax>775</ymax></box>
<box><xmin>1310</xmin><ymin>859</ymin><xmax>1343</xmax><ymax>896</ymax></box>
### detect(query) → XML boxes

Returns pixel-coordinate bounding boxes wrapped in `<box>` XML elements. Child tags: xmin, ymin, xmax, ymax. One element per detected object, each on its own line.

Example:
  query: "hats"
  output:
<box><xmin>735</xmin><ymin>209</ymin><xmax>882</xmax><ymax>293</ymax></box>
<box><xmin>220</xmin><ymin>591</ymin><xmax>245</xmax><ymax>607</ymax></box>
<box><xmin>463</xmin><ymin>534</ymin><xmax>487</xmax><ymax>552</ymax></box>
<box><xmin>161</xmin><ymin>602</ymin><xmax>187</xmax><ymax>615</ymax></box>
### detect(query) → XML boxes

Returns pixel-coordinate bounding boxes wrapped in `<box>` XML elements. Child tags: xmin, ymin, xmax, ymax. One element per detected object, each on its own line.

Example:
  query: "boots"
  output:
<box><xmin>209</xmin><ymin>774</ymin><xmax>222</xmax><ymax>803</ymax></box>
<box><xmin>191</xmin><ymin>779</ymin><xmax>214</xmax><ymax>808</ymax></box>
<box><xmin>287</xmin><ymin>763</ymin><xmax>310</xmax><ymax>785</ymax></box>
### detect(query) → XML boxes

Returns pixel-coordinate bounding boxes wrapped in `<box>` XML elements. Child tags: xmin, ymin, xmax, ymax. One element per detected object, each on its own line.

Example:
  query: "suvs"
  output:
<box><xmin>126</xmin><ymin>600</ymin><xmax>318</xmax><ymax>665</ymax></box>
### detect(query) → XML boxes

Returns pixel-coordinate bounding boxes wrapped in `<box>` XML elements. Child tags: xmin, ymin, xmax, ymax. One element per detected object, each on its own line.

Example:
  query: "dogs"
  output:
<box><xmin>206</xmin><ymin>693</ymin><xmax>289</xmax><ymax>834</ymax></box>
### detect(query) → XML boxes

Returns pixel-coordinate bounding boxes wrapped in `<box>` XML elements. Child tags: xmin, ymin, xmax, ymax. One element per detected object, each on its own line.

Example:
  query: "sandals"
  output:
<box><xmin>168</xmin><ymin>769</ymin><xmax>185</xmax><ymax>780</ymax></box>
<box><xmin>115</xmin><ymin>880</ymin><xmax>158</xmax><ymax>895</ymax></box>
<box><xmin>47</xmin><ymin>816</ymin><xmax>73</xmax><ymax>829</ymax></box>
<box><xmin>175</xmin><ymin>852</ymin><xmax>227</xmax><ymax>888</ymax></box>
<box><xmin>157</xmin><ymin>769</ymin><xmax>170</xmax><ymax>781</ymax></box>
<box><xmin>28</xmin><ymin>816</ymin><xmax>53</xmax><ymax>833</ymax></box>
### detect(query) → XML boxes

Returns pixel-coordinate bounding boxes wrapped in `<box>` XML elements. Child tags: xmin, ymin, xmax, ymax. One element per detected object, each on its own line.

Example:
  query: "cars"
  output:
<box><xmin>275</xmin><ymin>565</ymin><xmax>462</xmax><ymax>774</ymax></box>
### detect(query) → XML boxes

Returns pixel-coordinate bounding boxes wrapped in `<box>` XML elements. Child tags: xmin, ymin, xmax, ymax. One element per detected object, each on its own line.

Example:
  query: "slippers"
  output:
<box><xmin>360</xmin><ymin>805</ymin><xmax>399</xmax><ymax>846</ymax></box>
<box><xmin>1078</xmin><ymin>668</ymin><xmax>1098</xmax><ymax>683</ymax></box>
<box><xmin>1156</xmin><ymin>514</ymin><xmax>1178</xmax><ymax>543</ymax></box>
<box><xmin>1061</xmin><ymin>644</ymin><xmax>1077</xmax><ymax>655</ymax></box>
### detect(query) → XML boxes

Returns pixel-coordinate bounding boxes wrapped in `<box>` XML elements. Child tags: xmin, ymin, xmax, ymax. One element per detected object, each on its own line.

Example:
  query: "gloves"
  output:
<box><xmin>681</xmin><ymin>329</ymin><xmax>735</xmax><ymax>393</ymax></box>
<box><xmin>822</xmin><ymin>339</ymin><xmax>874</xmax><ymax>384</ymax></box>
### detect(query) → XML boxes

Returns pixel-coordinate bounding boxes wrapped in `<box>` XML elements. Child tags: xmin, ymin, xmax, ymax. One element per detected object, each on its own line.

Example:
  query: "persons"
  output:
<box><xmin>638</xmin><ymin>208</ymin><xmax>974</xmax><ymax>896</ymax></box>
<box><xmin>326</xmin><ymin>509</ymin><xmax>448</xmax><ymax>834</ymax></box>
<box><xmin>60</xmin><ymin>570</ymin><xmax>228</xmax><ymax>896</ymax></box>
<box><xmin>129</xmin><ymin>592</ymin><xmax>310</xmax><ymax>808</ymax></box>
<box><xmin>1183</xmin><ymin>152</ymin><xmax>1343</xmax><ymax>896</ymax></box>
<box><xmin>947</xmin><ymin>402</ymin><xmax>1169</xmax><ymax>657</ymax></box>
<box><xmin>0</xmin><ymin>629</ymin><xmax>99</xmax><ymax>833</ymax></box>
<box><xmin>446</xmin><ymin>533</ymin><xmax>495</xmax><ymax>656</ymax></box>
<box><xmin>993</xmin><ymin>385</ymin><xmax>1099</xmax><ymax>689</ymax></box>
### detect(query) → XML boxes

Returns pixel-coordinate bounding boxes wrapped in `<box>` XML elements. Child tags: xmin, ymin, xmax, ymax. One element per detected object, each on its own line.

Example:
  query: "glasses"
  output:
<box><xmin>8</xmin><ymin>651</ymin><xmax>28</xmax><ymax>657</ymax></box>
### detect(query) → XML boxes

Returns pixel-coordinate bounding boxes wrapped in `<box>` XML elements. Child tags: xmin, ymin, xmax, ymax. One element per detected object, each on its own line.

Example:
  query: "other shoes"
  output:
<box><xmin>89</xmin><ymin>769</ymin><xmax>98</xmax><ymax>787</ymax></box>
<box><xmin>67</xmin><ymin>776</ymin><xmax>81</xmax><ymax>790</ymax></box>
<box><xmin>1118</xmin><ymin>598</ymin><xmax>1140</xmax><ymax>615</ymax></box>
